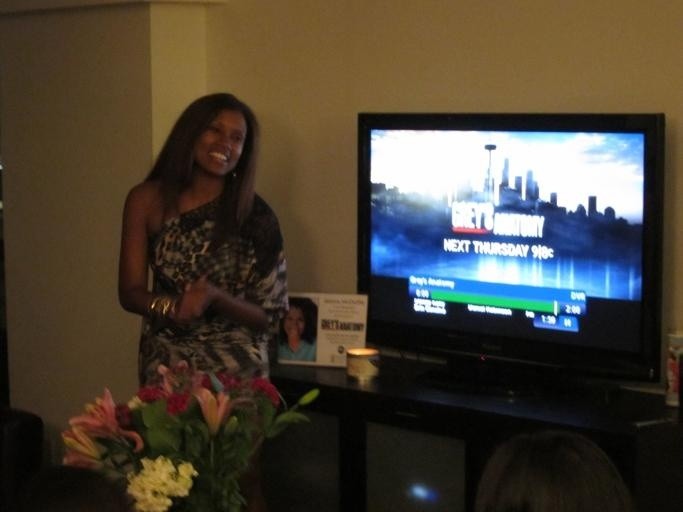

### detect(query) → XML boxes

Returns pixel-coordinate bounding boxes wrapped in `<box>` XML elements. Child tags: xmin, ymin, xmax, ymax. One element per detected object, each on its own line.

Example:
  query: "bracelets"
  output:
<box><xmin>145</xmin><ymin>295</ymin><xmax>179</xmax><ymax>320</ymax></box>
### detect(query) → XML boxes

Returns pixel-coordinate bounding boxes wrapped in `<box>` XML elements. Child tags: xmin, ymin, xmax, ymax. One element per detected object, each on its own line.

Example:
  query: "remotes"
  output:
<box><xmin>591</xmin><ymin>383</ymin><xmax>620</xmax><ymax>408</ymax></box>
<box><xmin>629</xmin><ymin>417</ymin><xmax>673</xmax><ymax>432</ymax></box>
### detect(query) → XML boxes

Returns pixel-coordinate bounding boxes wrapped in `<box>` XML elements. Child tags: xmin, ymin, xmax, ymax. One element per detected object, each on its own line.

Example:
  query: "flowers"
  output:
<box><xmin>60</xmin><ymin>359</ymin><xmax>321</xmax><ymax>512</ymax></box>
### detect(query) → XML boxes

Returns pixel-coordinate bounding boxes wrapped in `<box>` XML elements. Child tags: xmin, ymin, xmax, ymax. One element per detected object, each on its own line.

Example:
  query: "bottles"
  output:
<box><xmin>665</xmin><ymin>332</ymin><xmax>682</xmax><ymax>408</ymax></box>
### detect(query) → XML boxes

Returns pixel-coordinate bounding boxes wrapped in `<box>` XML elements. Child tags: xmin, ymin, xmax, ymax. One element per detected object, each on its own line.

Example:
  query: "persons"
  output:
<box><xmin>268</xmin><ymin>296</ymin><xmax>317</xmax><ymax>363</ymax></box>
<box><xmin>117</xmin><ymin>92</ymin><xmax>289</xmax><ymax>391</ymax></box>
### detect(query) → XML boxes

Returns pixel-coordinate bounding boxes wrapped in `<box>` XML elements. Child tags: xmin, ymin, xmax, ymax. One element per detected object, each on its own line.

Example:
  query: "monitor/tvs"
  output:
<box><xmin>358</xmin><ymin>114</ymin><xmax>665</xmax><ymax>397</ymax></box>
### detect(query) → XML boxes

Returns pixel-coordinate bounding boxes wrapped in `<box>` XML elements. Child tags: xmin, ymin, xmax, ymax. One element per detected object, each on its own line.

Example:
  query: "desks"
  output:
<box><xmin>271</xmin><ymin>355</ymin><xmax>683</xmax><ymax>512</ymax></box>
